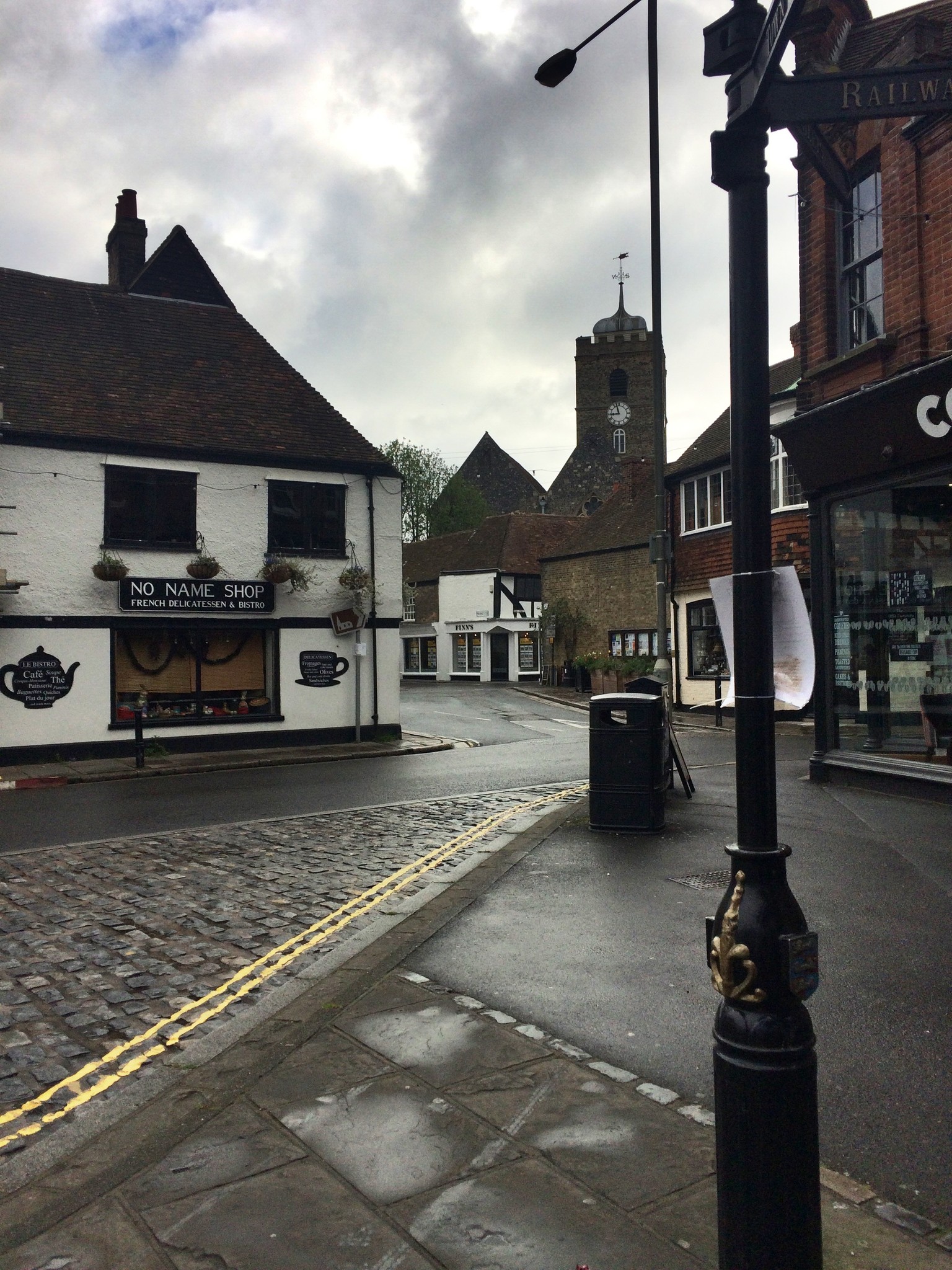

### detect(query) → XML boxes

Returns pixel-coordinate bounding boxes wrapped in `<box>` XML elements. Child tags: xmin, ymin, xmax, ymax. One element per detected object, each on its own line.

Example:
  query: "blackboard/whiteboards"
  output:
<box><xmin>542</xmin><ymin>664</ymin><xmax>550</xmax><ymax>680</ymax></box>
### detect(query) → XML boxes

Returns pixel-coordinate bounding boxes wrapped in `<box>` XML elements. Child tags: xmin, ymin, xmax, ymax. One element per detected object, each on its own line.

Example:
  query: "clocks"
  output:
<box><xmin>607</xmin><ymin>401</ymin><xmax>632</xmax><ymax>426</ymax></box>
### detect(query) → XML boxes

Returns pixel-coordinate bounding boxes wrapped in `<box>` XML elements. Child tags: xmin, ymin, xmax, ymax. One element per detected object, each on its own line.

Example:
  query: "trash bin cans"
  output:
<box><xmin>561</xmin><ymin>660</ymin><xmax>575</xmax><ymax>687</ymax></box>
<box><xmin>588</xmin><ymin>693</ymin><xmax>671</xmax><ymax>835</ymax></box>
<box><xmin>575</xmin><ymin>665</ymin><xmax>592</xmax><ymax>693</ymax></box>
<box><xmin>624</xmin><ymin>675</ymin><xmax>674</xmax><ymax>788</ymax></box>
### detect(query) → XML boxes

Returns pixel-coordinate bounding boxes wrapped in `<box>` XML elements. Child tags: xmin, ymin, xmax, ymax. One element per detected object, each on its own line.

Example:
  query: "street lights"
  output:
<box><xmin>529</xmin><ymin>0</ymin><xmax>674</xmax><ymax>759</ymax></box>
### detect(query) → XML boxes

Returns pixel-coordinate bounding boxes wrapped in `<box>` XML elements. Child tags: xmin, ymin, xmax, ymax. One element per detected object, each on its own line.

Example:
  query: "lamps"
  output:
<box><xmin>490</xmin><ymin>585</ymin><xmax>493</xmax><ymax>593</ymax></box>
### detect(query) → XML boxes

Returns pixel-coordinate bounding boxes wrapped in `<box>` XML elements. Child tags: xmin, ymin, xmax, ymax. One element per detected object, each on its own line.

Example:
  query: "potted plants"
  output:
<box><xmin>333</xmin><ymin>565</ymin><xmax>385</xmax><ymax>613</ymax></box>
<box><xmin>92</xmin><ymin>547</ymin><xmax>128</xmax><ymax>581</ymax></box>
<box><xmin>572</xmin><ymin>653</ymin><xmax>653</xmax><ymax>695</ymax></box>
<box><xmin>255</xmin><ymin>553</ymin><xmax>322</xmax><ymax>592</ymax></box>
<box><xmin>186</xmin><ymin>556</ymin><xmax>220</xmax><ymax>579</ymax></box>
